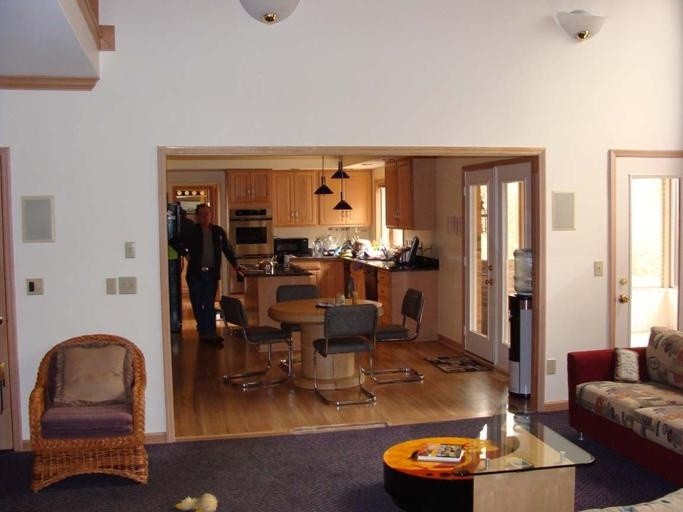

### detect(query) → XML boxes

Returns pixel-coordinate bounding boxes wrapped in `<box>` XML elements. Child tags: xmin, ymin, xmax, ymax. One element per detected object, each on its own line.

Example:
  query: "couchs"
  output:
<box><xmin>567</xmin><ymin>326</ymin><xmax>683</xmax><ymax>485</ymax></box>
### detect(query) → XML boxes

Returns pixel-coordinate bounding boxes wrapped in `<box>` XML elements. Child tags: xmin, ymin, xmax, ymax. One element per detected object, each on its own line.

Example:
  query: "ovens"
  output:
<box><xmin>228</xmin><ymin>208</ymin><xmax>273</xmax><ymax>294</ymax></box>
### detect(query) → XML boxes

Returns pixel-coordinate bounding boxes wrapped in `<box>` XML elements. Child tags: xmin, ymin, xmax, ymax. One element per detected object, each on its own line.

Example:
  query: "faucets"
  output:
<box><xmin>269</xmin><ymin>254</ymin><xmax>277</xmax><ymax>263</ymax></box>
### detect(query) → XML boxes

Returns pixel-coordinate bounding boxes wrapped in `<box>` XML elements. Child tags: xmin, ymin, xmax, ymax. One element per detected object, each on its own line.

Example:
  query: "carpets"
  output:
<box><xmin>0</xmin><ymin>412</ymin><xmax>683</xmax><ymax>512</ymax></box>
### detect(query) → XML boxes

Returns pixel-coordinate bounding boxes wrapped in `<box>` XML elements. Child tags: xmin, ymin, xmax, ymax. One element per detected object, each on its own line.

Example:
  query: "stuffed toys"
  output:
<box><xmin>177</xmin><ymin>493</ymin><xmax>218</xmax><ymax>512</ymax></box>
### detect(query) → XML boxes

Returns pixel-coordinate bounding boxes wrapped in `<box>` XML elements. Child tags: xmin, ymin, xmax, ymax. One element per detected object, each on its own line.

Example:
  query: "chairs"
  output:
<box><xmin>29</xmin><ymin>334</ymin><xmax>150</xmax><ymax>491</ymax></box>
<box><xmin>220</xmin><ymin>285</ymin><xmax>426</xmax><ymax>406</ymax></box>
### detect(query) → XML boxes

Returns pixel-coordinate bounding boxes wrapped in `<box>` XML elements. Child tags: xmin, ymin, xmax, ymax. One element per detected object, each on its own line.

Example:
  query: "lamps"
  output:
<box><xmin>313</xmin><ymin>155</ymin><xmax>353</xmax><ymax>211</ymax></box>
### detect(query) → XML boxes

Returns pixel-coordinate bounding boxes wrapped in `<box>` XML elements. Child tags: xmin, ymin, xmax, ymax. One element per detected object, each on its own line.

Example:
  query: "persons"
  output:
<box><xmin>169</xmin><ymin>203</ymin><xmax>248</xmax><ymax>345</ymax></box>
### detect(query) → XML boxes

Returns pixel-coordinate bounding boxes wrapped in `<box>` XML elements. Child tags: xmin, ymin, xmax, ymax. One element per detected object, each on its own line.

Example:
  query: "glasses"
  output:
<box><xmin>196</xmin><ymin>212</ymin><xmax>211</xmax><ymax>217</ymax></box>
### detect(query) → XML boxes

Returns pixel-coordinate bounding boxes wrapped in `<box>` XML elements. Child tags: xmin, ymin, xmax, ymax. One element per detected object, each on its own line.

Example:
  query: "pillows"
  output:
<box><xmin>614</xmin><ymin>347</ymin><xmax>640</xmax><ymax>381</ymax></box>
<box><xmin>646</xmin><ymin>326</ymin><xmax>683</xmax><ymax>388</ymax></box>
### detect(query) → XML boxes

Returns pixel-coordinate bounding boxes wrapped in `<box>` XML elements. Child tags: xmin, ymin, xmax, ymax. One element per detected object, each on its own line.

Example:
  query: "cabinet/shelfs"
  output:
<box><xmin>272</xmin><ymin>170</ymin><xmax>319</xmax><ymax>226</ymax></box>
<box><xmin>225</xmin><ymin>169</ymin><xmax>272</xmax><ymax>210</ymax></box>
<box><xmin>319</xmin><ymin>169</ymin><xmax>374</xmax><ymax>227</ymax></box>
<box><xmin>376</xmin><ymin>269</ymin><xmax>439</xmax><ymax>342</ymax></box>
<box><xmin>385</xmin><ymin>157</ymin><xmax>435</xmax><ymax>230</ymax></box>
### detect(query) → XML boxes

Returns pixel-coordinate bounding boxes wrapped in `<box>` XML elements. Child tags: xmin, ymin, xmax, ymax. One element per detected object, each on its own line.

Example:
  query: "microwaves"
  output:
<box><xmin>274</xmin><ymin>237</ymin><xmax>308</xmax><ymax>257</ymax></box>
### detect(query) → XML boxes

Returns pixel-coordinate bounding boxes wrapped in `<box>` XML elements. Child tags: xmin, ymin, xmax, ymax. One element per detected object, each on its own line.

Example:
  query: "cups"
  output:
<box><xmin>351</xmin><ymin>290</ymin><xmax>358</xmax><ymax>304</ymax></box>
<box><xmin>334</xmin><ymin>293</ymin><xmax>344</xmax><ymax>305</ymax></box>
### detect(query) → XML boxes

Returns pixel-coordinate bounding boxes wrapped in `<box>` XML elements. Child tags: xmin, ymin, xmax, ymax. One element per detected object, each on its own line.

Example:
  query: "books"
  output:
<box><xmin>416</xmin><ymin>444</ymin><xmax>464</xmax><ymax>463</ymax></box>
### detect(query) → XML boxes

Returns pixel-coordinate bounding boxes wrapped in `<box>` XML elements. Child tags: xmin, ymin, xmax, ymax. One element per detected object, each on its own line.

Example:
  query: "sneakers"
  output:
<box><xmin>199</xmin><ymin>332</ymin><xmax>224</xmax><ymax>345</ymax></box>
